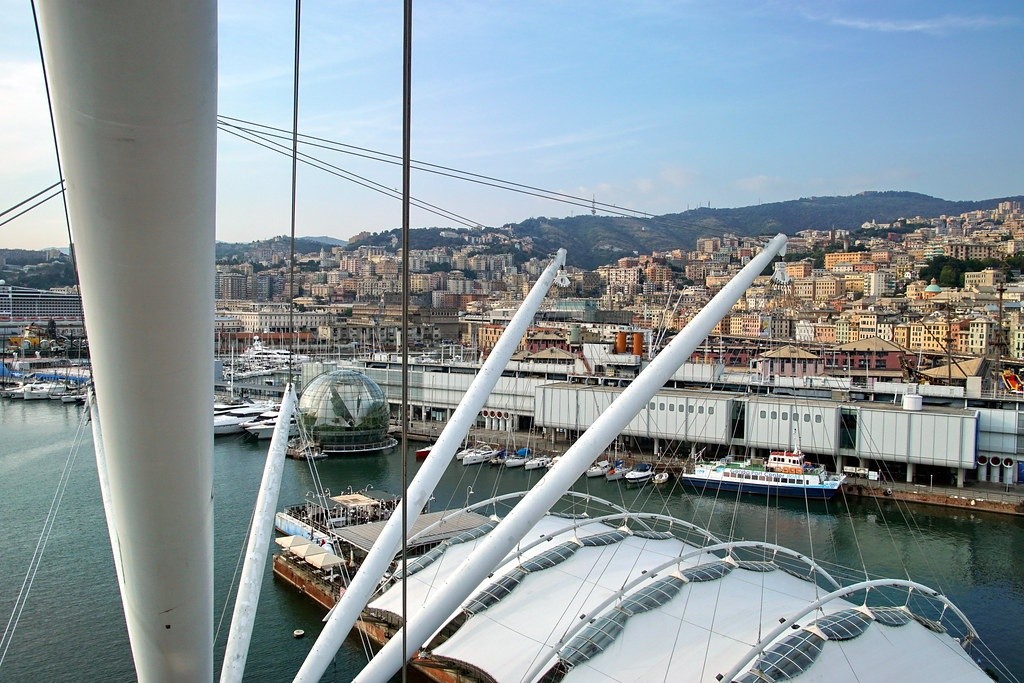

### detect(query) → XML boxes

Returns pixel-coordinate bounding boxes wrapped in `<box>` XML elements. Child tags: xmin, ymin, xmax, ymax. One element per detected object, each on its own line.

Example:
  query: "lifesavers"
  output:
<box><xmin>783</xmin><ymin>466</ymin><xmax>803</xmax><ymax>474</ymax></box>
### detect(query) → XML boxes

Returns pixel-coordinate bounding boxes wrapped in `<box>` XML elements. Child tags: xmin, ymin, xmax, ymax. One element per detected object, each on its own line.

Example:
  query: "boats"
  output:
<box><xmin>505</xmin><ymin>447</ymin><xmax>532</xmax><ymax>468</ymax></box>
<box><xmin>415</xmin><ymin>445</ymin><xmax>434</xmax><ymax>458</ymax></box>
<box><xmin>545</xmin><ymin>455</ymin><xmax>561</xmax><ymax>471</ymax></box>
<box><xmin>652</xmin><ymin>472</ymin><xmax>670</xmax><ymax>484</ymax></box>
<box><xmin>0</xmin><ymin>336</ymin><xmax>88</xmax><ymax>403</ymax></box>
<box><xmin>682</xmin><ymin>441</ymin><xmax>846</xmax><ymax>499</ymax></box>
<box><xmin>606</xmin><ymin>459</ymin><xmax>630</xmax><ymax>482</ymax></box>
<box><xmin>525</xmin><ymin>455</ymin><xmax>551</xmax><ymax>470</ymax></box>
<box><xmin>586</xmin><ymin>459</ymin><xmax>610</xmax><ymax>478</ymax></box>
<box><xmin>456</xmin><ymin>440</ymin><xmax>501</xmax><ymax>466</ymax></box>
<box><xmin>286</xmin><ymin>436</ymin><xmax>328</xmax><ymax>460</ymax></box>
<box><xmin>488</xmin><ymin>448</ymin><xmax>513</xmax><ymax>466</ymax></box>
<box><xmin>213</xmin><ymin>336</ymin><xmax>356</xmax><ymax>439</ymax></box>
<box><xmin>624</xmin><ymin>462</ymin><xmax>656</xmax><ymax>484</ymax></box>
